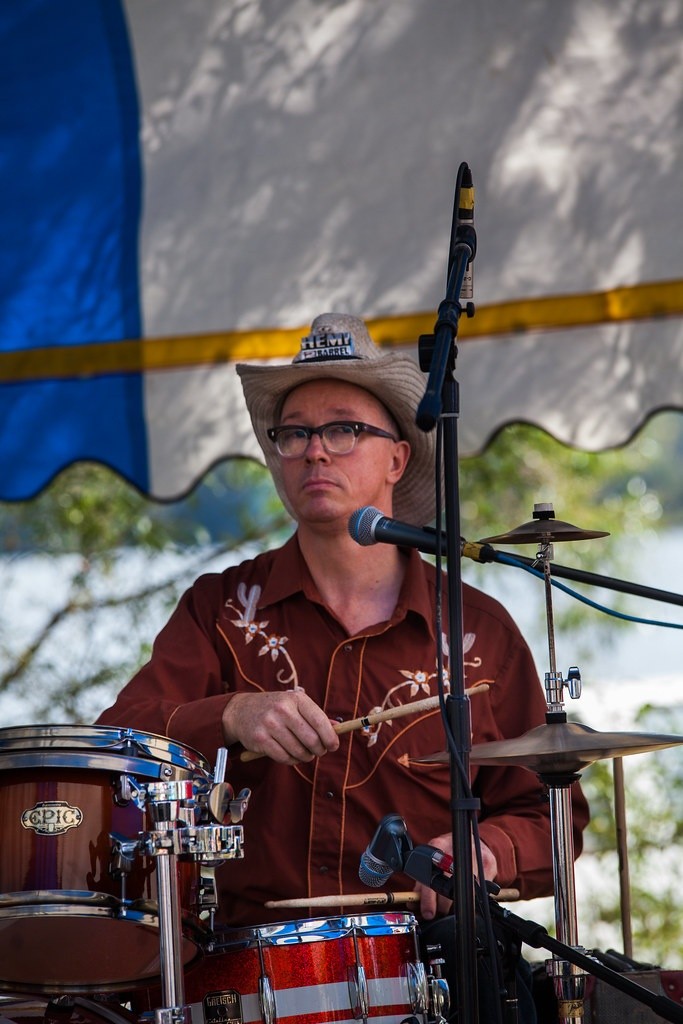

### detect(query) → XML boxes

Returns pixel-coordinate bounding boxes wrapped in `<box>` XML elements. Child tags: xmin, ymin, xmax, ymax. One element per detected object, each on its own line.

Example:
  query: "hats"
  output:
<box><xmin>235</xmin><ymin>313</ymin><xmax>446</xmax><ymax>527</ymax></box>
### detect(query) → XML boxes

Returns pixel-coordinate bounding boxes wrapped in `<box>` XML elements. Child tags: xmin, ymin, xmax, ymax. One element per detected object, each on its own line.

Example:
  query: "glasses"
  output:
<box><xmin>266</xmin><ymin>419</ymin><xmax>399</xmax><ymax>459</ymax></box>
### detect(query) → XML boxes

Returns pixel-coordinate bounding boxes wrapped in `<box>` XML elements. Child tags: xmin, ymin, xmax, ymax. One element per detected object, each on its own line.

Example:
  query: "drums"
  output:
<box><xmin>2</xmin><ymin>724</ymin><xmax>251</xmax><ymax>1001</ymax></box>
<box><xmin>185</xmin><ymin>913</ymin><xmax>454</xmax><ymax>1024</ymax></box>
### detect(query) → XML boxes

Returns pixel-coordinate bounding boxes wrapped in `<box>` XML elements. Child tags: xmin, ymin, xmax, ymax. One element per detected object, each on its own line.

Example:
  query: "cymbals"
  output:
<box><xmin>476</xmin><ymin>514</ymin><xmax>609</xmax><ymax>545</ymax></box>
<box><xmin>414</xmin><ymin>724</ymin><xmax>683</xmax><ymax>768</ymax></box>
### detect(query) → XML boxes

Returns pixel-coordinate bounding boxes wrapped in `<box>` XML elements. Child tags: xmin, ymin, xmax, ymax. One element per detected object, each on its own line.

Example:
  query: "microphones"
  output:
<box><xmin>456</xmin><ymin>162</ymin><xmax>475</xmax><ymax>299</ymax></box>
<box><xmin>347</xmin><ymin>505</ymin><xmax>494</xmax><ymax>563</ymax></box>
<box><xmin>358</xmin><ymin>813</ymin><xmax>408</xmax><ymax>888</ymax></box>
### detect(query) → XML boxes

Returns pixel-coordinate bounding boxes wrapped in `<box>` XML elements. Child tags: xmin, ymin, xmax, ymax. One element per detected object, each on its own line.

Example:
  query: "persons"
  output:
<box><xmin>94</xmin><ymin>315</ymin><xmax>591</xmax><ymax>1024</ymax></box>
